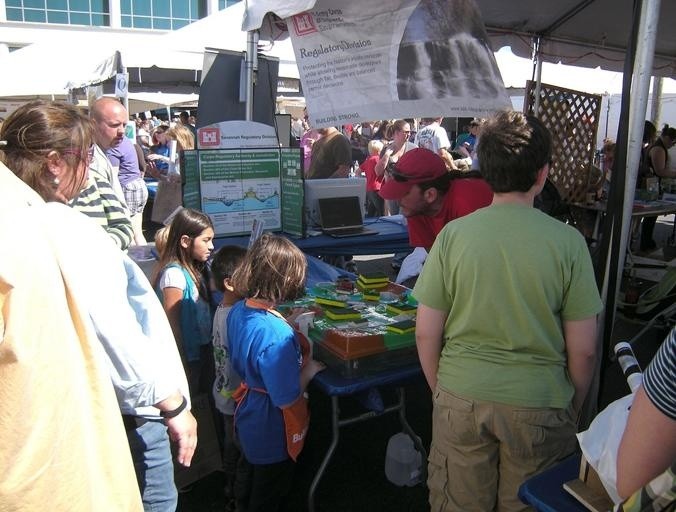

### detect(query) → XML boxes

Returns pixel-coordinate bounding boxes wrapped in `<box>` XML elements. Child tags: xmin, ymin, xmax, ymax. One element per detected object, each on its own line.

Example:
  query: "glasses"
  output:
<box><xmin>52</xmin><ymin>144</ymin><xmax>96</xmax><ymax>164</ymax></box>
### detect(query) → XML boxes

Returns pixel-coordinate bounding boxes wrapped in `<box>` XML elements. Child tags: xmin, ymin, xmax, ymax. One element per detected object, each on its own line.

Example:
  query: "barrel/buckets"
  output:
<box><xmin>384</xmin><ymin>427</ymin><xmax>423</xmax><ymax>487</ymax></box>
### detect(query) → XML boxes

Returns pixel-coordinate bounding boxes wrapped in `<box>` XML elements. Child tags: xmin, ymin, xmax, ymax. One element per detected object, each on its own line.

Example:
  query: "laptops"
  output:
<box><xmin>317</xmin><ymin>196</ymin><xmax>379</xmax><ymax>238</ymax></box>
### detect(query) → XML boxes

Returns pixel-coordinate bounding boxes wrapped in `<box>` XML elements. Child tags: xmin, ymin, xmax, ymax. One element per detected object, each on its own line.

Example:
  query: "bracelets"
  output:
<box><xmin>160</xmin><ymin>398</ymin><xmax>193</xmax><ymax>417</ymax></box>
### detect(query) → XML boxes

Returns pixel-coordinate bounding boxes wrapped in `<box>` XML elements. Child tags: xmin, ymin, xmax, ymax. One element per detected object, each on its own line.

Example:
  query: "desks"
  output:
<box><xmin>572</xmin><ymin>198</ymin><xmax>675</xmax><ymax>250</ymax></box>
<box><xmin>209</xmin><ymin>251</ymin><xmax>430</xmax><ymax>512</ymax></box>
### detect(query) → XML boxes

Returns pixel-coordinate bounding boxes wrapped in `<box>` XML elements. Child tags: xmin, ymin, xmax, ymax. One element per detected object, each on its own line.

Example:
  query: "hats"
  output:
<box><xmin>377</xmin><ymin>149</ymin><xmax>446</xmax><ymax>201</ymax></box>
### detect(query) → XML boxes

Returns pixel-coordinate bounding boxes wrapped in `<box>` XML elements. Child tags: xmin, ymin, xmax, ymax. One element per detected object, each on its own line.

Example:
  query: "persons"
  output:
<box><xmin>377</xmin><ymin>150</ymin><xmax>495</xmax><ymax>261</ymax></box>
<box><xmin>293</xmin><ymin>117</ymin><xmax>301</xmax><ymax>137</ymax></box>
<box><xmin>102</xmin><ymin>135</ymin><xmax>150</xmax><ymax>247</ymax></box>
<box><xmin>374</xmin><ymin>121</ymin><xmax>420</xmax><ymax>216</ymax></box>
<box><xmin>136</xmin><ymin>120</ymin><xmax>153</xmax><ymax>160</ymax></box>
<box><xmin>65</xmin><ymin>158</ymin><xmax>133</xmax><ymax>253</ymax></box>
<box><xmin>84</xmin><ymin>97</ymin><xmax>131</xmax><ymax>235</ymax></box>
<box><xmin>404</xmin><ymin>118</ymin><xmax>419</xmax><ymax>144</ymax></box>
<box><xmin>453</xmin><ymin>122</ymin><xmax>473</xmax><ymax>156</ymax></box>
<box><xmin>132</xmin><ymin>116</ymin><xmax>143</xmax><ymax>131</ymax></box>
<box><xmin>159</xmin><ymin>207</ymin><xmax>216</xmax><ymax>499</ymax></box>
<box><xmin>301</xmin><ymin>113</ymin><xmax>328</xmax><ymax>178</ymax></box>
<box><xmin>0</xmin><ymin>100</ymin><xmax>199</xmax><ymax>512</ymax></box>
<box><xmin>632</xmin><ymin>120</ymin><xmax>658</xmax><ymax>240</ymax></box>
<box><xmin>211</xmin><ymin>247</ymin><xmax>257</xmax><ymax>498</ymax></box>
<box><xmin>134</xmin><ymin>125</ymin><xmax>172</xmax><ymax>184</ymax></box>
<box><xmin>355</xmin><ymin>140</ymin><xmax>387</xmax><ymax>218</ymax></box>
<box><xmin>224</xmin><ymin>237</ymin><xmax>323</xmax><ymax>503</ymax></box>
<box><xmin>148</xmin><ymin>128</ymin><xmax>197</xmax><ymax>193</ymax></box>
<box><xmin>290</xmin><ymin>117</ymin><xmax>297</xmax><ymax>148</ymax></box>
<box><xmin>148</xmin><ymin>115</ymin><xmax>164</xmax><ymax>128</ymax></box>
<box><xmin>301</xmin><ymin>119</ymin><xmax>309</xmax><ymax>140</ymax></box>
<box><xmin>419</xmin><ymin>116</ymin><xmax>458</xmax><ymax>171</ymax></box>
<box><xmin>414</xmin><ymin>112</ymin><xmax>602</xmax><ymax>509</ymax></box>
<box><xmin>359</xmin><ymin>121</ymin><xmax>377</xmax><ymax>140</ymax></box>
<box><xmin>306</xmin><ymin>124</ymin><xmax>351</xmax><ymax>179</ymax></box>
<box><xmin>616</xmin><ymin>324</ymin><xmax>674</xmax><ymax>507</ymax></box>
<box><xmin>459</xmin><ymin>121</ymin><xmax>480</xmax><ymax>161</ymax></box>
<box><xmin>154</xmin><ymin>226</ymin><xmax>179</xmax><ymax>311</ymax></box>
<box><xmin>642</xmin><ymin>126</ymin><xmax>675</xmax><ymax>250</ymax></box>
<box><xmin>597</xmin><ymin>139</ymin><xmax>618</xmax><ymax>191</ymax></box>
<box><xmin>178</xmin><ymin>112</ymin><xmax>197</xmax><ymax>138</ymax></box>
<box><xmin>376</xmin><ymin>120</ymin><xmax>397</xmax><ymax>161</ymax></box>
